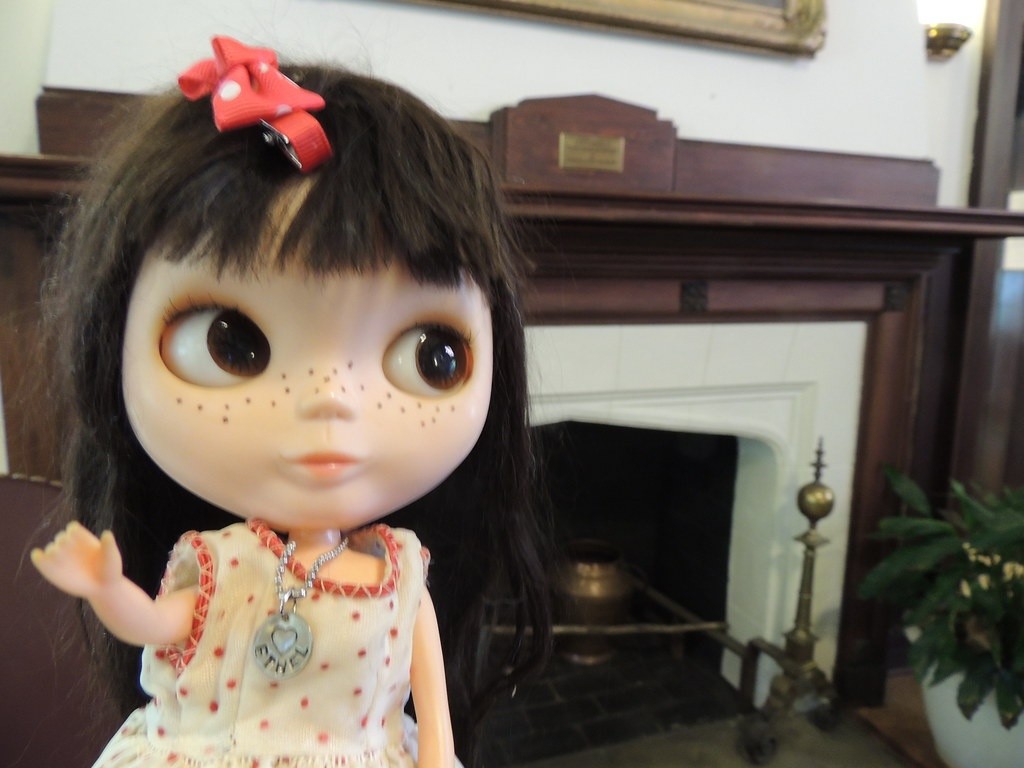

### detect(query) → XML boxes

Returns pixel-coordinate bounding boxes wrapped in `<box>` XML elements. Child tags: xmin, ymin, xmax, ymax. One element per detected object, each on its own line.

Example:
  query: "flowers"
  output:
<box><xmin>851</xmin><ymin>459</ymin><xmax>1024</xmax><ymax>728</ymax></box>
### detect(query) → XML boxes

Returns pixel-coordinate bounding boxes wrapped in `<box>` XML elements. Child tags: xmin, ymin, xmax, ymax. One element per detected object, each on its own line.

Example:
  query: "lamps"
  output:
<box><xmin>917</xmin><ymin>0</ymin><xmax>983</xmax><ymax>62</ymax></box>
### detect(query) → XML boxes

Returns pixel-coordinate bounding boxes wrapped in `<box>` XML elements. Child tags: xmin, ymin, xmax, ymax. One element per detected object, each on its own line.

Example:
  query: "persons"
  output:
<box><xmin>28</xmin><ymin>36</ymin><xmax>555</xmax><ymax>768</ymax></box>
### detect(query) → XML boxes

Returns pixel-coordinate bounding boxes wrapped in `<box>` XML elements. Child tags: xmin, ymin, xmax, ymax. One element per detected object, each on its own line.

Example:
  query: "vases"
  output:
<box><xmin>905</xmin><ymin>626</ymin><xmax>1024</xmax><ymax>768</ymax></box>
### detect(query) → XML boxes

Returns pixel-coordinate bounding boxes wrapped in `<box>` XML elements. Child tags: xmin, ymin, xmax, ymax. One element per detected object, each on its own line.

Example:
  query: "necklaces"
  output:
<box><xmin>249</xmin><ymin>537</ymin><xmax>349</xmax><ymax>679</ymax></box>
